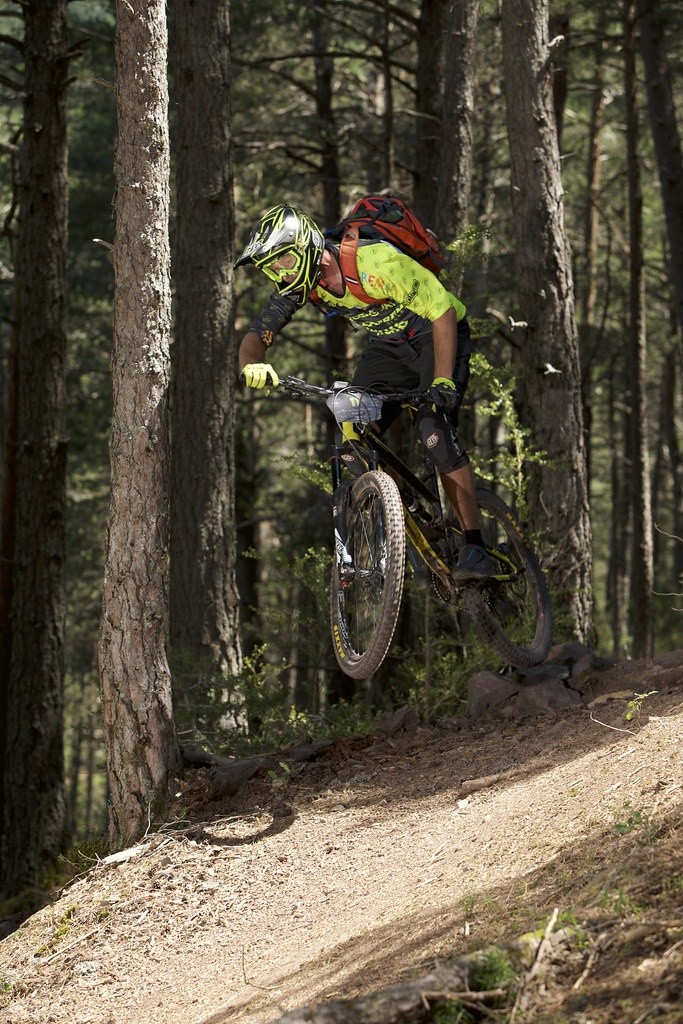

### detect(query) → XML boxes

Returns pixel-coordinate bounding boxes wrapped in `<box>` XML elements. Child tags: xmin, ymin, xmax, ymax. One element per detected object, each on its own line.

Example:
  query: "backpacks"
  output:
<box><xmin>308</xmin><ymin>195</ymin><xmax>442</xmax><ymax>306</ymax></box>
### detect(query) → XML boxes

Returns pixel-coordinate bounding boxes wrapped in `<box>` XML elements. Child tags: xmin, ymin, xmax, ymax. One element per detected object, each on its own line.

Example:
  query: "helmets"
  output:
<box><xmin>232</xmin><ymin>202</ymin><xmax>326</xmax><ymax>306</ymax></box>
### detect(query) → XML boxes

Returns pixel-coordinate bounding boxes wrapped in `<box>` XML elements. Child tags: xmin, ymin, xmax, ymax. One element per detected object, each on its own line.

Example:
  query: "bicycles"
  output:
<box><xmin>239</xmin><ymin>369</ymin><xmax>556</xmax><ymax>683</ymax></box>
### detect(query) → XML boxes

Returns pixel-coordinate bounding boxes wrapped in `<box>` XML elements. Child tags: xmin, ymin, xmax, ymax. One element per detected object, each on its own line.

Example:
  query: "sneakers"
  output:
<box><xmin>451</xmin><ymin>544</ymin><xmax>496</xmax><ymax>581</ymax></box>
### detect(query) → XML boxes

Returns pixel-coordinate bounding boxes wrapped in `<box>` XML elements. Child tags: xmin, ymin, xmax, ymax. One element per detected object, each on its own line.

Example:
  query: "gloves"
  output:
<box><xmin>241</xmin><ymin>363</ymin><xmax>279</xmax><ymax>390</ymax></box>
<box><xmin>426</xmin><ymin>377</ymin><xmax>457</xmax><ymax>415</ymax></box>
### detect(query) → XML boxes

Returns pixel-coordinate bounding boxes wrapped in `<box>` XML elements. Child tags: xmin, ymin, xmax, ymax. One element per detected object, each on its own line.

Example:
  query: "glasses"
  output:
<box><xmin>258</xmin><ymin>246</ymin><xmax>306</xmax><ymax>283</ymax></box>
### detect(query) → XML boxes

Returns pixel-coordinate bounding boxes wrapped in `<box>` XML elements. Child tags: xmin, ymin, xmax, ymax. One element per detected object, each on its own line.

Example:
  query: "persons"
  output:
<box><xmin>238</xmin><ymin>201</ymin><xmax>498</xmax><ymax>580</ymax></box>
